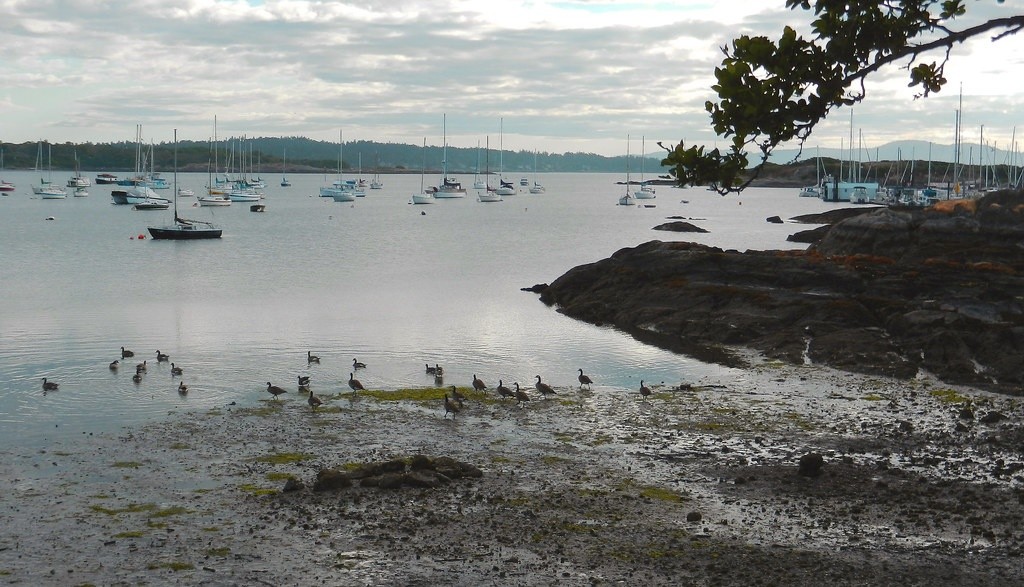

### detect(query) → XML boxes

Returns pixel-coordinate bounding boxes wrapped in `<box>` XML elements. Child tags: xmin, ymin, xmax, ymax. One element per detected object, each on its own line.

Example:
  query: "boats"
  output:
<box><xmin>520</xmin><ymin>178</ymin><xmax>531</xmax><ymax>186</ymax></box>
<box><xmin>94</xmin><ymin>173</ymin><xmax>118</xmax><ymax>184</ymax></box>
<box><xmin>0</xmin><ymin>184</ymin><xmax>16</xmax><ymax>193</ymax></box>
<box><xmin>425</xmin><ymin>184</ymin><xmax>439</xmax><ymax>195</ymax></box>
<box><xmin>176</xmin><ymin>188</ymin><xmax>196</xmax><ymax>198</ymax></box>
<box><xmin>249</xmin><ymin>205</ymin><xmax>267</xmax><ymax>213</ymax></box>
<box><xmin>1</xmin><ymin>180</ymin><xmax>12</xmax><ymax>186</ymax></box>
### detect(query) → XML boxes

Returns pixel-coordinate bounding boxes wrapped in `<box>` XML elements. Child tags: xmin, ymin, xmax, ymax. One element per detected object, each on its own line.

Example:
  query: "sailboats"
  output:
<box><xmin>146</xmin><ymin>128</ymin><xmax>223</xmax><ymax>238</ymax></box>
<box><xmin>39</xmin><ymin>143</ymin><xmax>68</xmax><ymax>198</ymax></box>
<box><xmin>527</xmin><ymin>147</ymin><xmax>546</xmax><ymax>194</ymax></box>
<box><xmin>196</xmin><ymin>115</ymin><xmax>268</xmax><ymax>207</ymax></box>
<box><xmin>492</xmin><ymin>116</ymin><xmax>519</xmax><ymax>196</ymax></box>
<box><xmin>477</xmin><ymin>136</ymin><xmax>503</xmax><ymax>202</ymax></box>
<box><xmin>411</xmin><ymin>136</ymin><xmax>438</xmax><ymax>203</ymax></box>
<box><xmin>618</xmin><ymin>133</ymin><xmax>635</xmax><ymax>205</ymax></box>
<box><xmin>279</xmin><ymin>147</ymin><xmax>292</xmax><ymax>187</ymax></box>
<box><xmin>63</xmin><ymin>148</ymin><xmax>93</xmax><ymax>198</ymax></box>
<box><xmin>798</xmin><ymin>80</ymin><xmax>1024</xmax><ymax>204</ymax></box>
<box><xmin>431</xmin><ymin>113</ymin><xmax>467</xmax><ymax>198</ymax></box>
<box><xmin>635</xmin><ymin>135</ymin><xmax>656</xmax><ymax>199</ymax></box>
<box><xmin>368</xmin><ymin>147</ymin><xmax>384</xmax><ymax>190</ymax></box>
<box><xmin>110</xmin><ymin>122</ymin><xmax>173</xmax><ymax>211</ymax></box>
<box><xmin>471</xmin><ymin>140</ymin><xmax>488</xmax><ymax>190</ymax></box>
<box><xmin>29</xmin><ymin>137</ymin><xmax>60</xmax><ymax>194</ymax></box>
<box><xmin>318</xmin><ymin>129</ymin><xmax>367</xmax><ymax>201</ymax></box>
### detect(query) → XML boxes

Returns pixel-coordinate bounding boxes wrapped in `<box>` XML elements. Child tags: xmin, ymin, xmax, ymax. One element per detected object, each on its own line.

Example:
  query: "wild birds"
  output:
<box><xmin>352</xmin><ymin>358</ymin><xmax>367</xmax><ymax>370</ymax></box>
<box><xmin>297</xmin><ymin>376</ymin><xmax>310</xmax><ymax>386</ymax></box>
<box><xmin>266</xmin><ymin>382</ymin><xmax>287</xmax><ymax>400</ymax></box>
<box><xmin>640</xmin><ymin>380</ymin><xmax>651</xmax><ymax>402</ymax></box>
<box><xmin>42</xmin><ymin>378</ymin><xmax>59</xmax><ymax>391</ymax></box>
<box><xmin>443</xmin><ymin>393</ymin><xmax>460</xmax><ymax>420</ymax></box>
<box><xmin>136</xmin><ymin>361</ymin><xmax>146</xmax><ymax>372</ymax></box>
<box><xmin>120</xmin><ymin>347</ymin><xmax>134</xmax><ymax>357</ymax></box>
<box><xmin>308</xmin><ymin>391</ymin><xmax>323</xmax><ymax>409</ymax></box>
<box><xmin>534</xmin><ymin>375</ymin><xmax>557</xmax><ymax>399</ymax></box>
<box><xmin>577</xmin><ymin>368</ymin><xmax>593</xmax><ymax>390</ymax></box>
<box><xmin>307</xmin><ymin>350</ymin><xmax>321</xmax><ymax>363</ymax></box>
<box><xmin>133</xmin><ymin>370</ymin><xmax>142</xmax><ymax>383</ymax></box>
<box><xmin>425</xmin><ymin>364</ymin><xmax>443</xmax><ymax>378</ymax></box>
<box><xmin>170</xmin><ymin>363</ymin><xmax>183</xmax><ymax>375</ymax></box>
<box><xmin>109</xmin><ymin>360</ymin><xmax>120</xmax><ymax>370</ymax></box>
<box><xmin>156</xmin><ymin>350</ymin><xmax>169</xmax><ymax>361</ymax></box>
<box><xmin>348</xmin><ymin>373</ymin><xmax>365</xmax><ymax>397</ymax></box>
<box><xmin>179</xmin><ymin>381</ymin><xmax>188</xmax><ymax>392</ymax></box>
<box><xmin>473</xmin><ymin>374</ymin><xmax>530</xmax><ymax>407</ymax></box>
